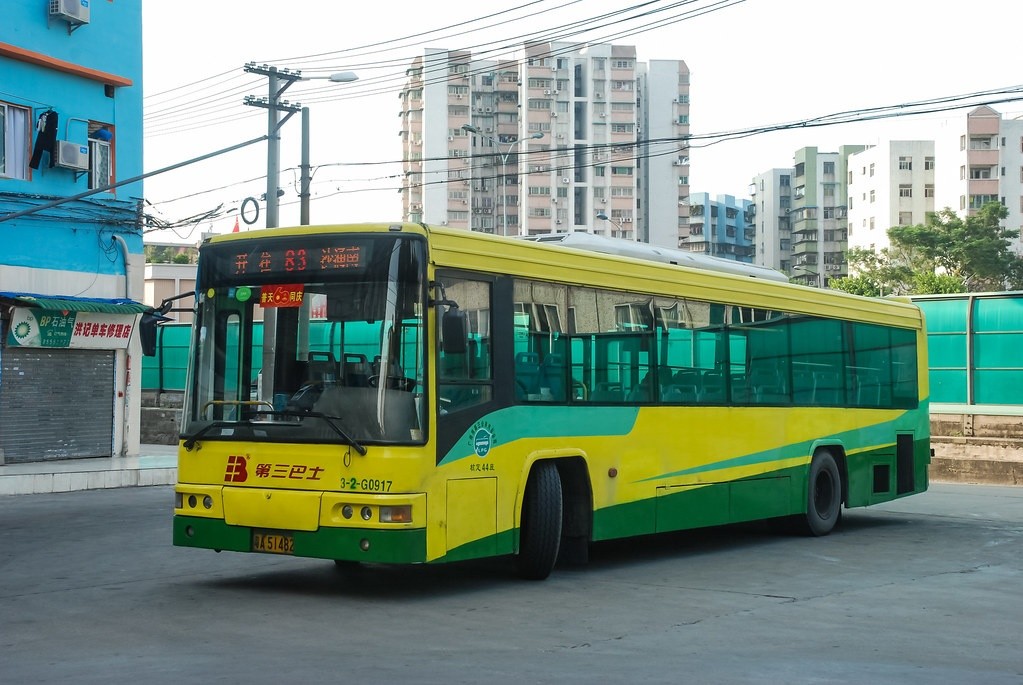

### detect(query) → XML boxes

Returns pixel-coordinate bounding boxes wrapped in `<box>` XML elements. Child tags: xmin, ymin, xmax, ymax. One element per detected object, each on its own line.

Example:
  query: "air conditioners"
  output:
<box><xmin>49</xmin><ymin>117</ymin><xmax>92</xmax><ymax>173</ymax></box>
<box><xmin>46</xmin><ymin>0</ymin><xmax>91</xmax><ymax>36</ymax></box>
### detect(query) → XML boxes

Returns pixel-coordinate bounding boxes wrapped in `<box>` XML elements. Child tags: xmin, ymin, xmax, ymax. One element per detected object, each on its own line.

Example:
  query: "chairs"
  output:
<box><xmin>298</xmin><ymin>337</ymin><xmax>903</xmax><ymax>414</ymax></box>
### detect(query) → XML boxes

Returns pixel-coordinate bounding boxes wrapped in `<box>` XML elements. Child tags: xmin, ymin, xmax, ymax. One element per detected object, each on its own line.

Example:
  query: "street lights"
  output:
<box><xmin>243</xmin><ymin>61</ymin><xmax>359</xmax><ymax>226</ymax></box>
<box><xmin>460</xmin><ymin>122</ymin><xmax>544</xmax><ymax>235</ymax></box>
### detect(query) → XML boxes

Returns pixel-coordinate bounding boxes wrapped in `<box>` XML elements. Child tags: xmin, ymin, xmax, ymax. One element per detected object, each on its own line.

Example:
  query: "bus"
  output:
<box><xmin>141</xmin><ymin>222</ymin><xmax>935</xmax><ymax>597</ymax></box>
